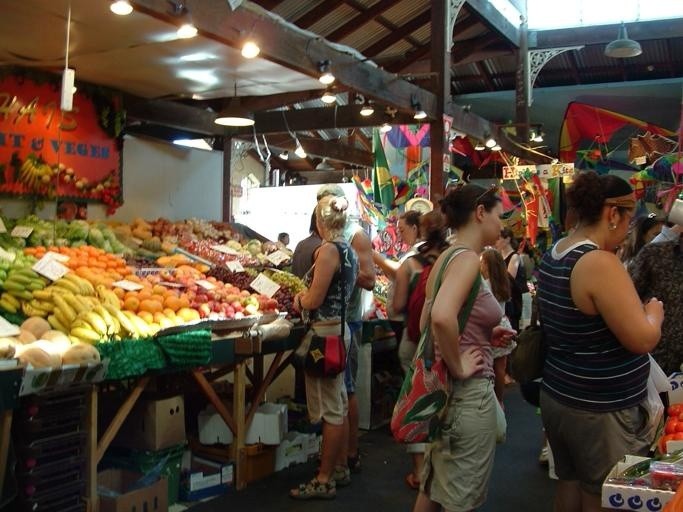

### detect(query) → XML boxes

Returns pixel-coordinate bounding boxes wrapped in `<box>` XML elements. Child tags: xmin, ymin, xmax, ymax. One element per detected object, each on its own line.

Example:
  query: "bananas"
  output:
<box><xmin>20</xmin><ymin>158</ymin><xmax>54</xmax><ymax>192</ymax></box>
<box><xmin>0</xmin><ymin>268</ymin><xmax>156</xmax><ymax>343</ymax></box>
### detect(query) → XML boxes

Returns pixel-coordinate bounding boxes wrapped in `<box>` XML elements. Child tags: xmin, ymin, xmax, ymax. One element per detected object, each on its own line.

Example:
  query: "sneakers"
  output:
<box><xmin>406</xmin><ymin>473</ymin><xmax>420</xmax><ymax>489</ymax></box>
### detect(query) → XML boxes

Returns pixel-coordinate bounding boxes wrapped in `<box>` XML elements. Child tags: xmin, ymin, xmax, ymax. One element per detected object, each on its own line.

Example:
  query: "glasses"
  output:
<box><xmin>474</xmin><ymin>184</ymin><xmax>498</xmax><ymax>208</ymax></box>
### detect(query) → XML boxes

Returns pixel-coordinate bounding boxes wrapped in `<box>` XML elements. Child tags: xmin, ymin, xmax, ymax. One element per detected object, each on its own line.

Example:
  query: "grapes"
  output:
<box><xmin>203</xmin><ymin>265</ymin><xmax>307</xmax><ymax>320</ymax></box>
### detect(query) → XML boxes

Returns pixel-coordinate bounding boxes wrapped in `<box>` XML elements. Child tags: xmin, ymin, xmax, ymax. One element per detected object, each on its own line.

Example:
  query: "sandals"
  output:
<box><xmin>311</xmin><ymin>466</ymin><xmax>350</xmax><ymax>487</ymax></box>
<box><xmin>348</xmin><ymin>455</ymin><xmax>360</xmax><ymax>471</ymax></box>
<box><xmin>289</xmin><ymin>478</ymin><xmax>336</xmax><ymax>499</ymax></box>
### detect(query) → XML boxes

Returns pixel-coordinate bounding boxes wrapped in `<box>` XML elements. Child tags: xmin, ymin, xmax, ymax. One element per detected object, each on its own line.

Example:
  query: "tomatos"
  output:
<box><xmin>661</xmin><ymin>403</ymin><xmax>683</xmax><ymax>452</ymax></box>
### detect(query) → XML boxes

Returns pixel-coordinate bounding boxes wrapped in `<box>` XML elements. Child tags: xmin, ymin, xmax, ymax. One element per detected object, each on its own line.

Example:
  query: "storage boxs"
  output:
<box><xmin>81</xmin><ymin>468</ymin><xmax>169</xmax><ymax>512</ymax></box>
<box><xmin>178</xmin><ymin>453</ymin><xmax>235</xmax><ymax>502</ymax></box>
<box><xmin>188</xmin><ymin>438</ymin><xmax>266</xmax><ymax>482</ymax></box>
<box><xmin>127</xmin><ymin>392</ymin><xmax>186</xmax><ymax>451</ymax></box>
<box><xmin>197</xmin><ymin>401</ymin><xmax>289</xmax><ymax>445</ymax></box>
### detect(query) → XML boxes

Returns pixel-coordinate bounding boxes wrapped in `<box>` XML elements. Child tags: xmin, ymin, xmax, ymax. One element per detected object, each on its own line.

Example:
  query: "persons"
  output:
<box><xmin>480</xmin><ymin>218</ymin><xmax>681</xmax><ymax>476</ymax></box>
<box><xmin>276</xmin><ymin>185</ymin><xmax>451</xmax><ymax>484</ymax></box>
<box><xmin>291</xmin><ymin>195</ymin><xmax>360</xmax><ymax>498</ymax></box>
<box><xmin>414</xmin><ymin>184</ymin><xmax>517</xmax><ymax>510</ymax></box>
<box><xmin>539</xmin><ymin>175</ymin><xmax>665</xmax><ymax>511</ymax></box>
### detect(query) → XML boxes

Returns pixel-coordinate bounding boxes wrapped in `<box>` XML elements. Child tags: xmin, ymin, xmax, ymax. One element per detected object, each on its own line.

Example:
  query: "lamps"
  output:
<box><xmin>475</xmin><ymin>143</ymin><xmax>484</xmax><ymax>150</ymax></box>
<box><xmin>295</xmin><ymin>147</ymin><xmax>306</xmax><ymax>159</ymax></box>
<box><xmin>604</xmin><ymin>20</ymin><xmax>643</xmax><ymax>58</ymax></box>
<box><xmin>493</xmin><ymin>145</ymin><xmax>503</xmax><ymax>151</ymax></box>
<box><xmin>321</xmin><ymin>91</ymin><xmax>337</xmax><ymax>104</ymax></box>
<box><xmin>413</xmin><ymin>104</ymin><xmax>428</xmax><ymax>119</ymax></box>
<box><xmin>279</xmin><ymin>151</ymin><xmax>291</xmax><ymax>160</ymax></box>
<box><xmin>214</xmin><ymin>97</ymin><xmax>255</xmax><ymax>126</ymax></box>
<box><xmin>110</xmin><ymin>2</ymin><xmax>132</xmax><ymax>17</ymax></box>
<box><xmin>487</xmin><ymin>138</ymin><xmax>496</xmax><ymax>148</ymax></box>
<box><xmin>319</xmin><ymin>68</ymin><xmax>336</xmax><ymax>85</ymax></box>
<box><xmin>174</xmin><ymin>138</ymin><xmax>213</xmax><ymax>151</ymax></box>
<box><xmin>529</xmin><ymin>132</ymin><xmax>542</xmax><ymax>142</ymax></box>
<box><xmin>178</xmin><ymin>14</ymin><xmax>198</xmax><ymax>40</ymax></box>
<box><xmin>241</xmin><ymin>35</ymin><xmax>260</xmax><ymax>60</ymax></box>
<box><xmin>360</xmin><ymin>103</ymin><xmax>374</xmax><ymax>117</ymax></box>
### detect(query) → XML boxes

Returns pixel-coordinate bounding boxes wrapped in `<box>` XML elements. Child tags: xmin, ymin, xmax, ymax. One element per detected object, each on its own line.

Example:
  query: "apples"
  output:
<box><xmin>367</xmin><ymin>297</ymin><xmax>387</xmax><ymax>320</ymax></box>
<box><xmin>146</xmin><ymin>268</ymin><xmax>288</xmax><ymax>320</ymax></box>
<box><xmin>43</xmin><ymin>163</ymin><xmax>111</xmax><ymax>194</ymax></box>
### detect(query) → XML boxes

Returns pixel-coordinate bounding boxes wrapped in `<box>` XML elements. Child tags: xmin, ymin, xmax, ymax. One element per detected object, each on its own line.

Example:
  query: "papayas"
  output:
<box><xmin>0</xmin><ymin>316</ymin><xmax>100</xmax><ymax>368</ymax></box>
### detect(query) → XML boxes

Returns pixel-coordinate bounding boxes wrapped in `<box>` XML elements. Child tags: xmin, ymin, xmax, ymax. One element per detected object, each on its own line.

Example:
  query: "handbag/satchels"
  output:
<box><xmin>515</xmin><ymin>265</ymin><xmax>529</xmax><ymax>293</ymax></box>
<box><xmin>303</xmin><ymin>335</ymin><xmax>347</xmax><ymax>379</ymax></box>
<box><xmin>392</xmin><ymin>325</ymin><xmax>452</xmax><ymax>444</ymax></box>
<box><xmin>403</xmin><ymin>254</ymin><xmax>433</xmax><ymax>338</ymax></box>
<box><xmin>512</xmin><ymin>325</ymin><xmax>544</xmax><ymax>380</ymax></box>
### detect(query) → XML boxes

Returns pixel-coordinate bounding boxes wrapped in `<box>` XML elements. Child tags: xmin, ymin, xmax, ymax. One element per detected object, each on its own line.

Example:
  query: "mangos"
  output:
<box><xmin>152</xmin><ymin>216</ymin><xmax>202</xmax><ymax>241</ymax></box>
<box><xmin>88</xmin><ymin>218</ymin><xmax>153</xmax><ymax>239</ymax></box>
<box><xmin>156</xmin><ymin>253</ymin><xmax>210</xmax><ymax>274</ymax></box>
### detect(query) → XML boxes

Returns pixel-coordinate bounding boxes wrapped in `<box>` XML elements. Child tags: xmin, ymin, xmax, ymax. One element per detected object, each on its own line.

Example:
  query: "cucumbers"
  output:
<box><xmin>619</xmin><ymin>456</ymin><xmax>680</xmax><ymax>477</ymax></box>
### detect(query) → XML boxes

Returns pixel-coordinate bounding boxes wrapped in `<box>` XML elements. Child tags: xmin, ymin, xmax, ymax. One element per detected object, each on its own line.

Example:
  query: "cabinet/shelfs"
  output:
<box><xmin>0</xmin><ymin>320</ymin><xmax>364</xmax><ymax>512</ymax></box>
